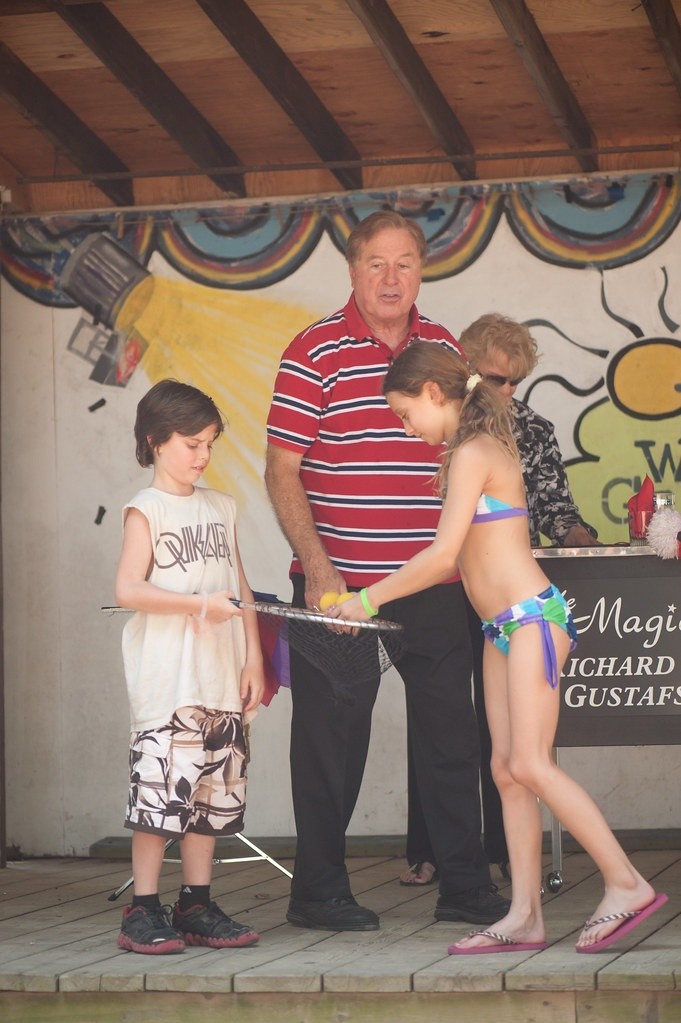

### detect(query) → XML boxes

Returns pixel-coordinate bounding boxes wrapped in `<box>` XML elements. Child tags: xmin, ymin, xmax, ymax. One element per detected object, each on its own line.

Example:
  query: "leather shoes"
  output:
<box><xmin>434</xmin><ymin>879</ymin><xmax>511</xmax><ymax>924</ymax></box>
<box><xmin>286</xmin><ymin>895</ymin><xmax>380</xmax><ymax>931</ymax></box>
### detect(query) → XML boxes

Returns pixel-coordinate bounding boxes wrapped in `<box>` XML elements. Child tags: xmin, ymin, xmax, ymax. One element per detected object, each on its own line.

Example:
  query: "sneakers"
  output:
<box><xmin>118</xmin><ymin>904</ymin><xmax>186</xmax><ymax>955</ymax></box>
<box><xmin>172</xmin><ymin>901</ymin><xmax>259</xmax><ymax>947</ymax></box>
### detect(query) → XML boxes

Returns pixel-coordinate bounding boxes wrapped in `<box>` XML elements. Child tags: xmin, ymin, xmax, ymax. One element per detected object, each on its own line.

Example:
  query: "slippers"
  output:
<box><xmin>400</xmin><ymin>858</ymin><xmax>440</xmax><ymax>886</ymax></box>
<box><xmin>448</xmin><ymin>928</ymin><xmax>546</xmax><ymax>954</ymax></box>
<box><xmin>575</xmin><ymin>891</ymin><xmax>669</xmax><ymax>953</ymax></box>
<box><xmin>500</xmin><ymin>853</ymin><xmax>513</xmax><ymax>882</ymax></box>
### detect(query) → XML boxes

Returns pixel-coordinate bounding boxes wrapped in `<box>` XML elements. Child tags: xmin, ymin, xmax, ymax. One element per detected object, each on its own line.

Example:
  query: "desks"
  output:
<box><xmin>530</xmin><ymin>546</ymin><xmax>681</xmax><ymax>893</ymax></box>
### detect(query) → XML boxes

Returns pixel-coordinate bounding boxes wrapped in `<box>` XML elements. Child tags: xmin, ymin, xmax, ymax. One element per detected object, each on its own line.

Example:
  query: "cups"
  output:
<box><xmin>628</xmin><ymin>511</ymin><xmax>652</xmax><ymax>546</ymax></box>
<box><xmin>653</xmin><ymin>492</ymin><xmax>676</xmax><ymax>513</ymax></box>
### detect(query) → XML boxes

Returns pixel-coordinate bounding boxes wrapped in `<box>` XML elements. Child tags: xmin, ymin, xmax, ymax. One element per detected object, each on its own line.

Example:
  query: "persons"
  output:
<box><xmin>115</xmin><ymin>379</ymin><xmax>266</xmax><ymax>954</ymax></box>
<box><xmin>401</xmin><ymin>313</ymin><xmax>601</xmax><ymax>888</ymax></box>
<box><xmin>263</xmin><ymin>209</ymin><xmax>511</xmax><ymax>930</ymax></box>
<box><xmin>326</xmin><ymin>339</ymin><xmax>668</xmax><ymax>953</ymax></box>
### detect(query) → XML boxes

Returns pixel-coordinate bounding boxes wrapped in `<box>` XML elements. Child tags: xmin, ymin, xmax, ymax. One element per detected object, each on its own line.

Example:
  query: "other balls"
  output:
<box><xmin>320</xmin><ymin>589</ymin><xmax>339</xmax><ymax>612</ymax></box>
<box><xmin>336</xmin><ymin>592</ymin><xmax>353</xmax><ymax>606</ymax></box>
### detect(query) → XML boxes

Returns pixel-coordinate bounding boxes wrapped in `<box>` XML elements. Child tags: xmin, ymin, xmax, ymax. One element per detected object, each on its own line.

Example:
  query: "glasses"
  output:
<box><xmin>476</xmin><ymin>368</ymin><xmax>526</xmax><ymax>386</ymax></box>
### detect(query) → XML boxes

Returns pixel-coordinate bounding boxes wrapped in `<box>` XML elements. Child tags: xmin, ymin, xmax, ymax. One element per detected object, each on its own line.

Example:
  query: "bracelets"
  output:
<box><xmin>359</xmin><ymin>587</ymin><xmax>379</xmax><ymax>617</ymax></box>
<box><xmin>199</xmin><ymin>591</ymin><xmax>208</xmax><ymax>622</ymax></box>
